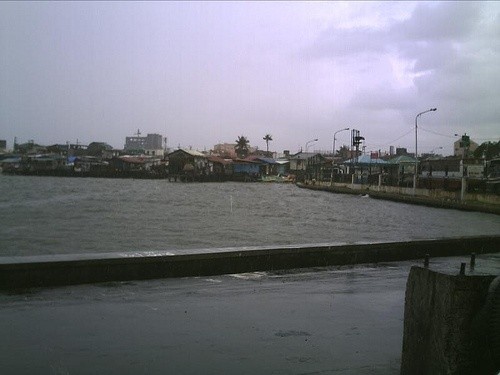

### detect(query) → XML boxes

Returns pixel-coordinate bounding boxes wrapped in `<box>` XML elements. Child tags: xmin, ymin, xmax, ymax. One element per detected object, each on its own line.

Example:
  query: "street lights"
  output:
<box><xmin>413</xmin><ymin>108</ymin><xmax>437</xmax><ymax>197</ymax></box>
<box><xmin>306</xmin><ymin>139</ymin><xmax>319</xmax><ymax>152</ymax></box>
<box><xmin>330</xmin><ymin>128</ymin><xmax>350</xmax><ymax>186</ymax></box>
